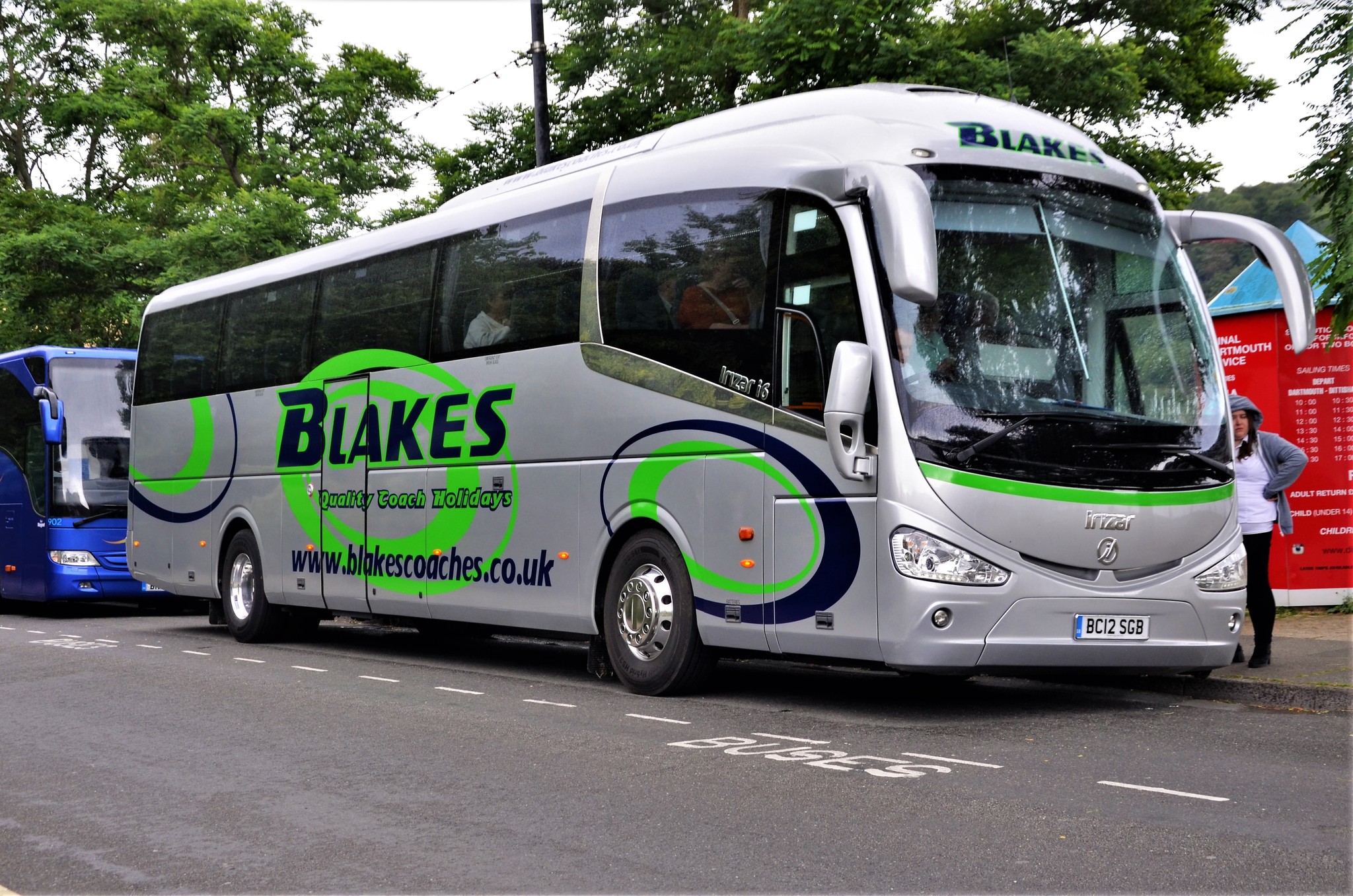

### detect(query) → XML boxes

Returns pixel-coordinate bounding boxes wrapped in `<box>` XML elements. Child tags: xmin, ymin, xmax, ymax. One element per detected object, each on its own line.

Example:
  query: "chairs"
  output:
<box><xmin>169</xmin><ymin>268</ymin><xmax>661</xmax><ymax>401</ymax></box>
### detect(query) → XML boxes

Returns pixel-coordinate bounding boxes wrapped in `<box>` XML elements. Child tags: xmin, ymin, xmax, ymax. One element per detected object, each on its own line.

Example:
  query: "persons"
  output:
<box><xmin>463</xmin><ymin>239</ymin><xmax>759</xmax><ymax>350</ymax></box>
<box><xmin>1228</xmin><ymin>394</ymin><xmax>1309</xmax><ymax>669</ymax></box>
<box><xmin>889</xmin><ymin>317</ymin><xmax>919</xmax><ymax>386</ymax></box>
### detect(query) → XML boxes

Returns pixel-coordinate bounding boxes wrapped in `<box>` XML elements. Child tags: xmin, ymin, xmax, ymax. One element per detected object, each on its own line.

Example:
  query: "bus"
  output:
<box><xmin>0</xmin><ymin>344</ymin><xmax>170</xmax><ymax>607</ymax></box>
<box><xmin>126</xmin><ymin>83</ymin><xmax>1315</xmax><ymax>693</ymax></box>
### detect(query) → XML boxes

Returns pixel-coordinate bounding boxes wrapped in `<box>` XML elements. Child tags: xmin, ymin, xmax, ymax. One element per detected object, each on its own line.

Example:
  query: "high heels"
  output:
<box><xmin>1248</xmin><ymin>647</ymin><xmax>1271</xmax><ymax>668</ymax></box>
<box><xmin>1233</xmin><ymin>642</ymin><xmax>1245</xmax><ymax>663</ymax></box>
